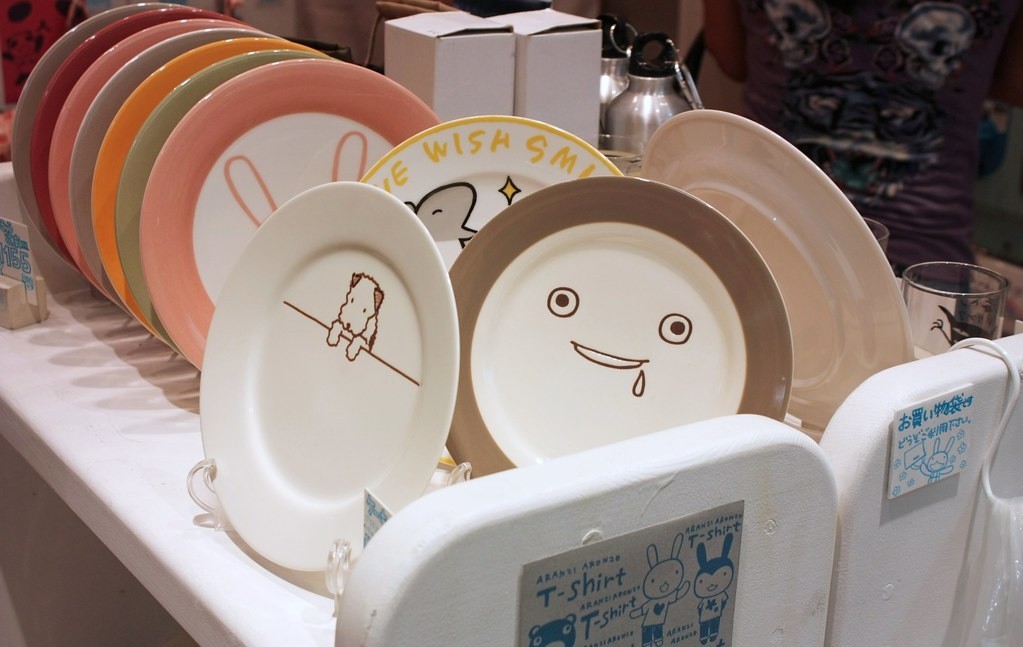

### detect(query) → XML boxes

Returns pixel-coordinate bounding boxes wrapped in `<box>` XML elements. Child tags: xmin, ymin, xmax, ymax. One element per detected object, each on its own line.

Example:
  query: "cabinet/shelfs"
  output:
<box><xmin>0</xmin><ymin>161</ymin><xmax>1023</xmax><ymax>647</ymax></box>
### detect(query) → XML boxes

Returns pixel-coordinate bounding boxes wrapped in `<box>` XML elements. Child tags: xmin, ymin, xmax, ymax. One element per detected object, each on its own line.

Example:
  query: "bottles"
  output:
<box><xmin>605</xmin><ymin>32</ymin><xmax>691</xmax><ymax>152</ymax></box>
<box><xmin>596</xmin><ymin>13</ymin><xmax>639</xmax><ymax>146</ymax></box>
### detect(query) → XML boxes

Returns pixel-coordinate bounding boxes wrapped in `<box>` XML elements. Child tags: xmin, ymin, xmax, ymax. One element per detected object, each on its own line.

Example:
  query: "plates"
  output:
<box><xmin>139</xmin><ymin>59</ymin><xmax>442</xmax><ymax>371</ymax></box>
<box><xmin>641</xmin><ymin>109</ymin><xmax>915</xmax><ymax>430</ymax></box>
<box><xmin>10</xmin><ymin>3</ymin><xmax>320</xmax><ymax>354</ymax></box>
<box><xmin>443</xmin><ymin>178</ymin><xmax>794</xmax><ymax>482</ymax></box>
<box><xmin>360</xmin><ymin>115</ymin><xmax>624</xmax><ymax>269</ymax></box>
<box><xmin>199</xmin><ymin>180</ymin><xmax>460</xmax><ymax>573</ymax></box>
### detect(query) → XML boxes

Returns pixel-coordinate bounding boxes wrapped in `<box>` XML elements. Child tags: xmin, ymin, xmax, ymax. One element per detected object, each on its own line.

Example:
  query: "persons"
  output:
<box><xmin>699</xmin><ymin>0</ymin><xmax>1023</xmax><ymax>323</ymax></box>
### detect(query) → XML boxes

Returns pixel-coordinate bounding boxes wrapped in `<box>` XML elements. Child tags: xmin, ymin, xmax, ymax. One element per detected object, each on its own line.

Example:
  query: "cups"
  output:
<box><xmin>862</xmin><ymin>217</ymin><xmax>888</xmax><ymax>255</ymax></box>
<box><xmin>903</xmin><ymin>261</ymin><xmax>1009</xmax><ymax>359</ymax></box>
